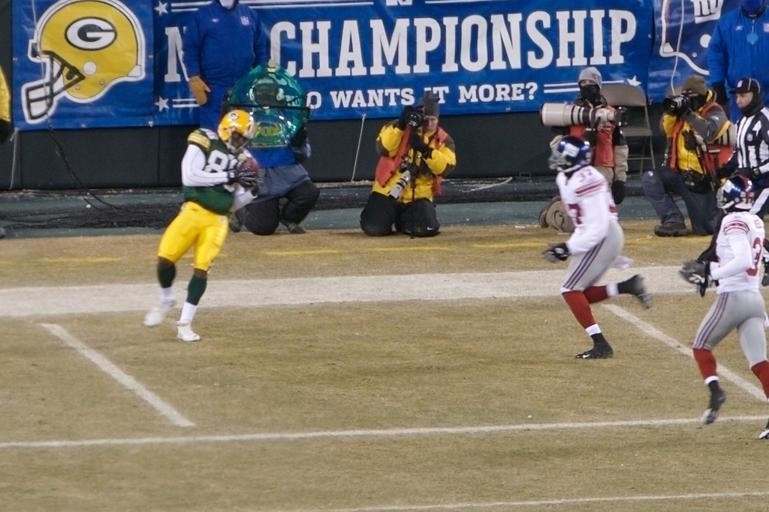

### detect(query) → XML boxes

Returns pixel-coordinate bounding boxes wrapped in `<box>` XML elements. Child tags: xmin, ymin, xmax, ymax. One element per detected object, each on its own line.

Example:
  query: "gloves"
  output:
<box><xmin>191</xmin><ymin>76</ymin><xmax>211</xmax><ymax>105</ymax></box>
<box><xmin>291</xmin><ymin>124</ymin><xmax>307</xmax><ymax>146</ymax></box>
<box><xmin>734</xmin><ymin>167</ymin><xmax>754</xmax><ymax>177</ymax></box>
<box><xmin>409</xmin><ymin>133</ymin><xmax>427</xmax><ymax>152</ymax></box>
<box><xmin>682</xmin><ymin>259</ymin><xmax>708</xmax><ymax>275</ymax></box>
<box><xmin>716</xmin><ymin>167</ymin><xmax>731</xmax><ymax>179</ymax></box>
<box><xmin>714</xmin><ymin>83</ymin><xmax>727</xmax><ymax>105</ymax></box>
<box><xmin>541</xmin><ymin>241</ymin><xmax>570</xmax><ymax>263</ymax></box>
<box><xmin>612</xmin><ymin>180</ymin><xmax>625</xmax><ymax>204</ymax></box>
<box><xmin>680</xmin><ymin>269</ymin><xmax>705</xmax><ymax>284</ymax></box>
<box><xmin>396</xmin><ymin>112</ymin><xmax>421</xmax><ymax>130</ymax></box>
<box><xmin>237</xmin><ymin>166</ymin><xmax>256</xmax><ymax>187</ymax></box>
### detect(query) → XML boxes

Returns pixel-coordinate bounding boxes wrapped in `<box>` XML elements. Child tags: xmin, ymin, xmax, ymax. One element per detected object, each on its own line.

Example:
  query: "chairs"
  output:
<box><xmin>602</xmin><ymin>85</ymin><xmax>656</xmax><ymax>177</ymax></box>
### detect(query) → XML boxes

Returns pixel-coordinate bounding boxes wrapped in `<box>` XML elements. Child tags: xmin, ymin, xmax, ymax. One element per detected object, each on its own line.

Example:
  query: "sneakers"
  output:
<box><xmin>143</xmin><ymin>296</ymin><xmax>177</xmax><ymax>326</ymax></box>
<box><xmin>628</xmin><ymin>275</ymin><xmax>652</xmax><ymax>309</ymax></box>
<box><xmin>279</xmin><ymin>214</ymin><xmax>305</xmax><ymax>233</ymax></box>
<box><xmin>576</xmin><ymin>346</ymin><xmax>613</xmax><ymax>359</ymax></box>
<box><xmin>702</xmin><ymin>391</ymin><xmax>726</xmax><ymax>424</ymax></box>
<box><xmin>653</xmin><ymin>222</ymin><xmax>689</xmax><ymax>236</ymax></box>
<box><xmin>229</xmin><ymin>212</ymin><xmax>242</xmax><ymax>232</ymax></box>
<box><xmin>177</xmin><ymin>324</ymin><xmax>200</xmax><ymax>342</ymax></box>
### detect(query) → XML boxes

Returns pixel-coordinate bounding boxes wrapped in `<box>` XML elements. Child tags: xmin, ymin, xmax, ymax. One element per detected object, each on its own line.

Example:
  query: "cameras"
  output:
<box><xmin>683</xmin><ymin>133</ymin><xmax>696</xmax><ymax>150</ymax></box>
<box><xmin>406</xmin><ymin>105</ymin><xmax>425</xmax><ymax>128</ymax></box>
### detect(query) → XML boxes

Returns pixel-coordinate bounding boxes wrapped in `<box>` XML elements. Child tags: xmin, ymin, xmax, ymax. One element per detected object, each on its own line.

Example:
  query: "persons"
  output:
<box><xmin>182</xmin><ymin>0</ymin><xmax>270</xmax><ymax>133</ymax></box>
<box><xmin>680</xmin><ymin>174</ymin><xmax>769</xmax><ymax>441</ymax></box>
<box><xmin>553</xmin><ymin>67</ymin><xmax>631</xmax><ymax>207</ymax></box>
<box><xmin>705</xmin><ymin>0</ymin><xmax>769</xmax><ymax>124</ymax></box>
<box><xmin>680</xmin><ymin>78</ymin><xmax>769</xmax><ymax>287</ymax></box>
<box><xmin>143</xmin><ymin>110</ymin><xmax>261</xmax><ymax>341</ymax></box>
<box><xmin>540</xmin><ymin>134</ymin><xmax>652</xmax><ymax>360</ymax></box>
<box><xmin>219</xmin><ymin>75</ymin><xmax>320</xmax><ymax>235</ymax></box>
<box><xmin>360</xmin><ymin>93</ymin><xmax>457</xmax><ymax>237</ymax></box>
<box><xmin>0</xmin><ymin>66</ymin><xmax>12</xmax><ymax>147</ymax></box>
<box><xmin>640</xmin><ymin>76</ymin><xmax>732</xmax><ymax>236</ymax></box>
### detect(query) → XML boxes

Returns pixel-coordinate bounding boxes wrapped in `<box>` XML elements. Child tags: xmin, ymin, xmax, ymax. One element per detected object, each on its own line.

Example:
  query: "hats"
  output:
<box><xmin>728</xmin><ymin>78</ymin><xmax>761</xmax><ymax>94</ymax></box>
<box><xmin>578</xmin><ymin>66</ymin><xmax>602</xmax><ymax>88</ymax></box>
<box><xmin>681</xmin><ymin>75</ymin><xmax>706</xmax><ymax>95</ymax></box>
<box><xmin>420</xmin><ymin>90</ymin><xmax>440</xmax><ymax>118</ymax></box>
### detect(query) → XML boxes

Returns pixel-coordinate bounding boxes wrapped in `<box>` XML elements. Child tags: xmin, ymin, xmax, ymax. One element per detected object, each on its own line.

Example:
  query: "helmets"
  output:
<box><xmin>548</xmin><ymin>135</ymin><xmax>592</xmax><ymax>173</ymax></box>
<box><xmin>217</xmin><ymin>109</ymin><xmax>258</xmax><ymax>155</ymax></box>
<box><xmin>716</xmin><ymin>176</ymin><xmax>755</xmax><ymax>209</ymax></box>
<box><xmin>250</xmin><ymin>76</ymin><xmax>279</xmax><ymax>102</ymax></box>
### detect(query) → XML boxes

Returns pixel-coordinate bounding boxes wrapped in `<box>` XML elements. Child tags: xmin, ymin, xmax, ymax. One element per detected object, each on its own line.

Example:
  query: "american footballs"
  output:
<box><xmin>242</xmin><ymin>157</ymin><xmax>258</xmax><ymax>178</ymax></box>
